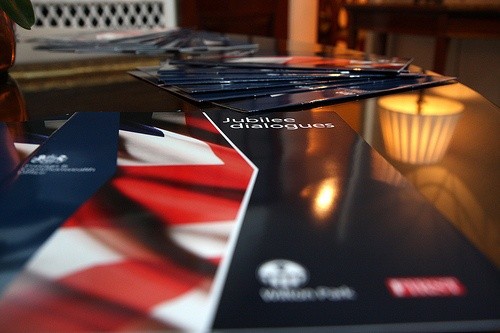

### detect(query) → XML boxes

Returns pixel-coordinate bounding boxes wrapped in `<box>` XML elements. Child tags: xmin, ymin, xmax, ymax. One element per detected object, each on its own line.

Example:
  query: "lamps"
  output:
<box><xmin>376</xmin><ymin>88</ymin><xmax>464</xmax><ymax>165</ymax></box>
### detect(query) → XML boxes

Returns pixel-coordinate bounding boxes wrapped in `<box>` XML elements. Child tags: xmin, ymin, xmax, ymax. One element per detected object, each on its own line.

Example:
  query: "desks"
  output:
<box><xmin>9</xmin><ymin>36</ymin><xmax>500</xmax><ymax>263</ymax></box>
